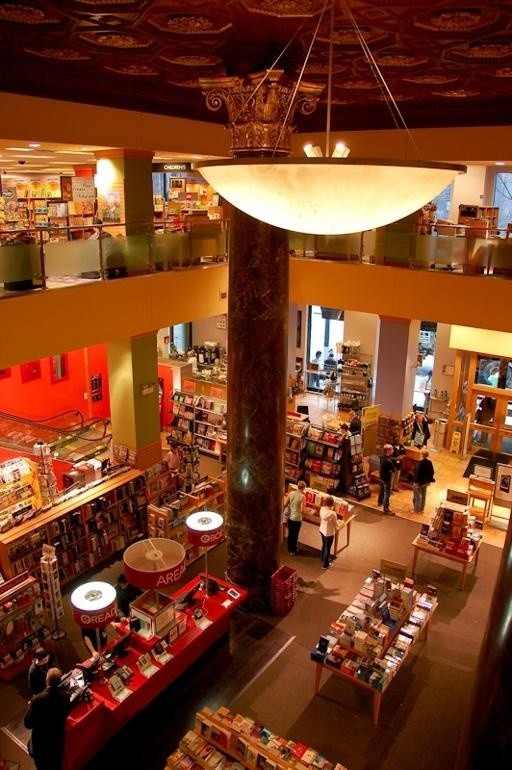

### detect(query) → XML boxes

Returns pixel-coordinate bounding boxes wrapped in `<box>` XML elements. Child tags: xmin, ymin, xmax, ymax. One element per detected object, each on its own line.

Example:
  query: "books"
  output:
<box><xmin>164</xmin><ymin>705</ymin><xmax>348</xmax><ymax>770</ymax></box>
<box><xmin>310</xmin><ymin>567</ymin><xmax>438</xmax><ymax>692</ymax></box>
<box><xmin>7</xmin><ymin>459</ymin><xmax>225</xmax><ymax>594</ymax></box>
<box><xmin>417</xmin><ymin>500</ymin><xmax>484</xmax><ymax>560</ymax></box>
<box><xmin>288</xmin><ymin>483</ymin><xmax>347</xmax><ymax>526</ymax></box>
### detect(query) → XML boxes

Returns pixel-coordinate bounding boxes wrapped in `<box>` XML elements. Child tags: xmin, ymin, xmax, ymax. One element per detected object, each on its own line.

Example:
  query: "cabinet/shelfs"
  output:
<box><xmin>422</xmin><ymin>201</ymin><xmax>500</xmax><ymax>276</ymax></box>
<box><xmin>0</xmin><ymin>175</ymin><xmax>222</xmax><ymax>259</ymax></box>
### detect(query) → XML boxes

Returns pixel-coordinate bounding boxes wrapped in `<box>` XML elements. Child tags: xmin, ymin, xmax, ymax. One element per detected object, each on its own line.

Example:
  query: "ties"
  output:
<box><xmin>419</xmin><ymin>423</ymin><xmax>424</xmax><ymax>430</ymax></box>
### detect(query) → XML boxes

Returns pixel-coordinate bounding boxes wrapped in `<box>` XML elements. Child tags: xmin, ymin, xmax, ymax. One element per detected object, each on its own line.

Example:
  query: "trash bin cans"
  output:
<box><xmin>271</xmin><ymin>565</ymin><xmax>298</xmax><ymax>616</ymax></box>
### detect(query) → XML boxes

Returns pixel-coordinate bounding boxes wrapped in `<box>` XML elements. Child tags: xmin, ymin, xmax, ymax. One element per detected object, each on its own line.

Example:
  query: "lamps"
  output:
<box><xmin>191</xmin><ymin>1</ymin><xmax>466</xmax><ymax>237</ymax></box>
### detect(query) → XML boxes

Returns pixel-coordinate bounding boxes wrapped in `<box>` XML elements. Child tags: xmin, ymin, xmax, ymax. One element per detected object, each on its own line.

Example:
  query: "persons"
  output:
<box><xmin>349</xmin><ymin>410</ymin><xmax>362</xmax><ymax>436</ymax></box>
<box><xmin>479</xmin><ymin>397</ymin><xmax>497</xmax><ymax>443</ymax></box>
<box><xmin>23</xmin><ymin>572</ymin><xmax>144</xmax><ymax>770</ymax></box>
<box><xmin>417</xmin><ymin>371</ymin><xmax>432</xmax><ymax>407</ymax></box>
<box><xmin>4</xmin><ymin>227</ymin><xmax>37</xmax><ymax>246</ymax></box>
<box><xmin>412</xmin><ymin>413</ymin><xmax>434</xmax><ymax>447</ymax></box>
<box><xmin>311</xmin><ymin>351</ymin><xmax>336</xmax><ymax>388</ymax></box>
<box><xmin>319</xmin><ymin>497</ymin><xmax>338</xmax><ymax>570</ymax></box>
<box><xmin>283</xmin><ymin>480</ymin><xmax>311</xmax><ymax>555</ymax></box>
<box><xmin>337</xmin><ymin>424</ymin><xmax>352</xmax><ymax>439</ymax></box>
<box><xmin>88</xmin><ymin>219</ymin><xmax>113</xmax><ymax>240</ymax></box>
<box><xmin>390</xmin><ymin>435</ymin><xmax>406</xmax><ymax>494</ymax></box>
<box><xmin>411</xmin><ymin>449</ymin><xmax>435</xmax><ymax>514</ymax></box>
<box><xmin>187</xmin><ymin>351</ymin><xmax>198</xmax><ymax>371</ymax></box>
<box><xmin>377</xmin><ymin>444</ymin><xmax>396</xmax><ymax>516</ymax></box>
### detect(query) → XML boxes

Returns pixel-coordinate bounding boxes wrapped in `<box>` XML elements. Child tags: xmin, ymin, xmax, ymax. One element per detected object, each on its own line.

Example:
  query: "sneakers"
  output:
<box><xmin>290</xmin><ymin>549</ymin><xmax>299</xmax><ymax>556</ymax></box>
<box><xmin>383</xmin><ymin>509</ymin><xmax>395</xmax><ymax>515</ymax></box>
<box><xmin>321</xmin><ymin>562</ymin><xmax>333</xmax><ymax>569</ymax></box>
